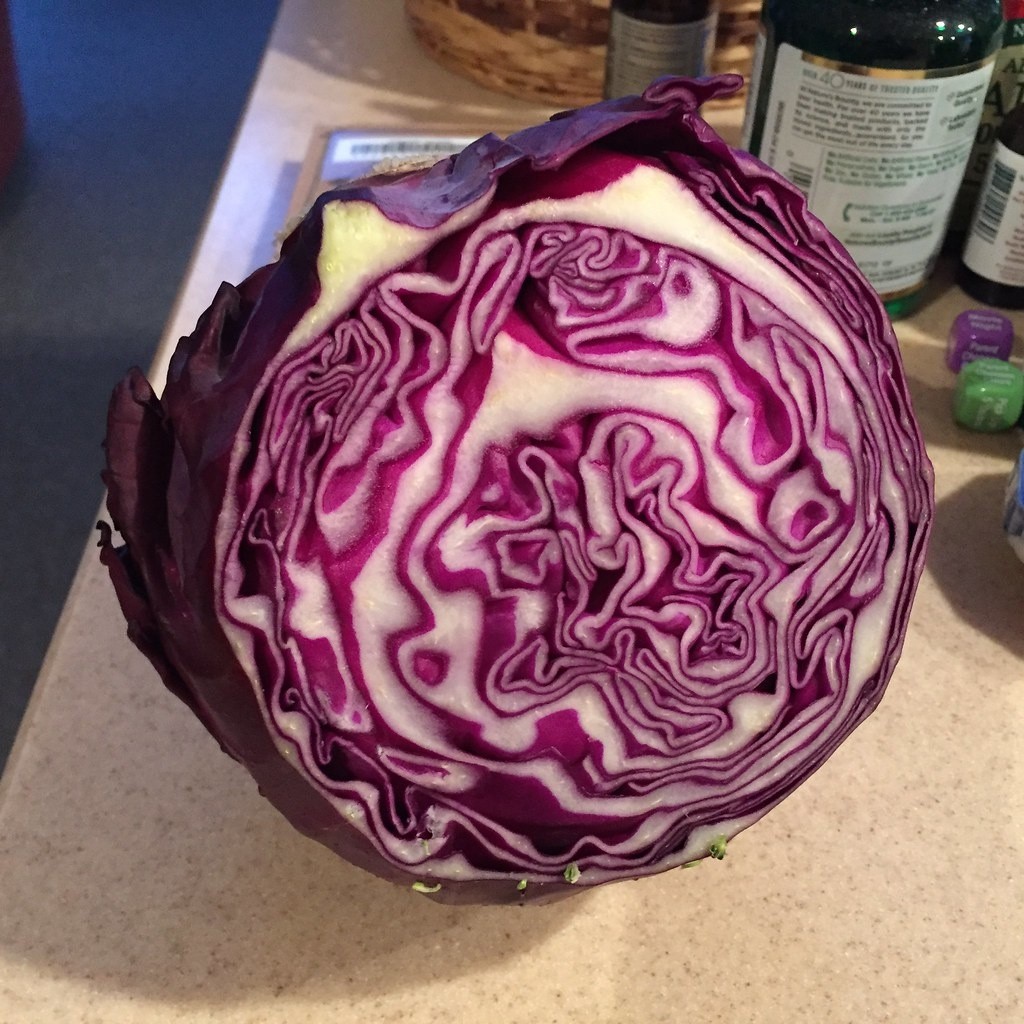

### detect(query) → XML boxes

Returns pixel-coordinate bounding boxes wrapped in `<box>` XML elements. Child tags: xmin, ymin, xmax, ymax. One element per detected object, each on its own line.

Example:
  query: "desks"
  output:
<box><xmin>0</xmin><ymin>0</ymin><xmax>1024</xmax><ymax>1024</ymax></box>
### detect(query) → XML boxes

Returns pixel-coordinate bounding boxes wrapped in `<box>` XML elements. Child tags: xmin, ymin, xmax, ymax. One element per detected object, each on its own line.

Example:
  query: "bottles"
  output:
<box><xmin>738</xmin><ymin>0</ymin><xmax>1024</xmax><ymax>321</ymax></box>
<box><xmin>604</xmin><ymin>0</ymin><xmax>719</xmax><ymax>103</ymax></box>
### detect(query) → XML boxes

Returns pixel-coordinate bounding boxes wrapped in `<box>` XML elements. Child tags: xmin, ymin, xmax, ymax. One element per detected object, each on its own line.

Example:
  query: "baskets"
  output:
<box><xmin>404</xmin><ymin>0</ymin><xmax>763</xmax><ymax>112</ymax></box>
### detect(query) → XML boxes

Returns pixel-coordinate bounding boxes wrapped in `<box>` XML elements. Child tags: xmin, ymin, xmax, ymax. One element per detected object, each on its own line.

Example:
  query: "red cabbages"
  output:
<box><xmin>102</xmin><ymin>75</ymin><xmax>933</xmax><ymax>880</ymax></box>
